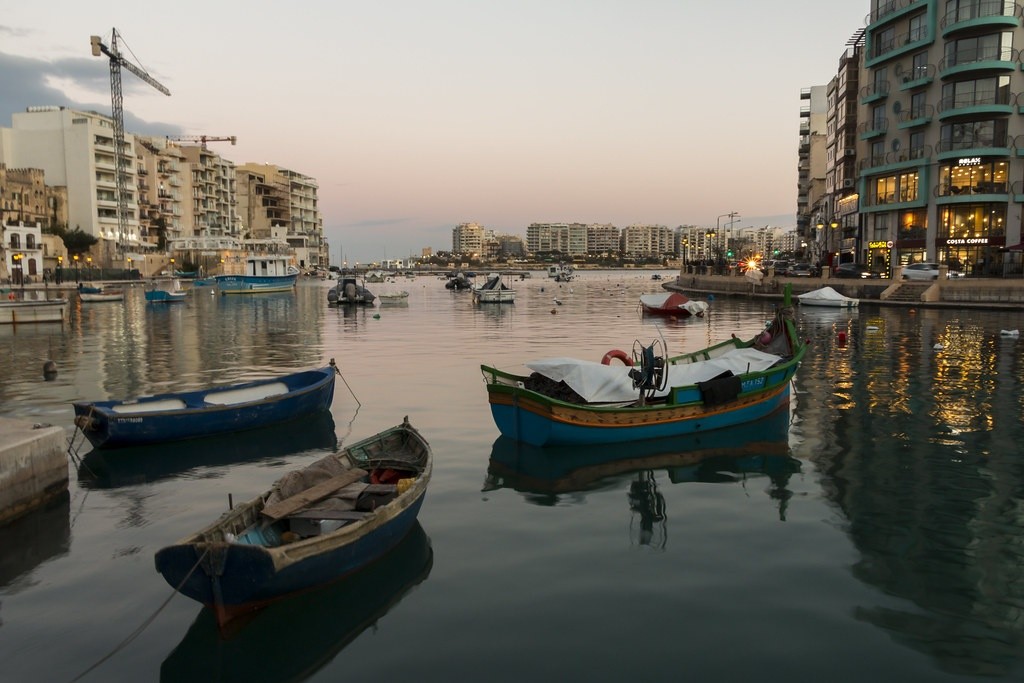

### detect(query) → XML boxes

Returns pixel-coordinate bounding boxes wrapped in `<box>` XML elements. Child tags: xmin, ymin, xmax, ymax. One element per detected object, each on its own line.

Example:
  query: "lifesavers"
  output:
<box><xmin>600</xmin><ymin>349</ymin><xmax>636</xmax><ymax>367</ymax></box>
<box><xmin>288</xmin><ymin>258</ymin><xmax>295</xmax><ymax>265</ymax></box>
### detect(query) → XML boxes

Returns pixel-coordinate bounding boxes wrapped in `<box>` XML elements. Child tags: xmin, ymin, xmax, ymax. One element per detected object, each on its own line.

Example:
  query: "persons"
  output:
<box><xmin>55</xmin><ymin>262</ymin><xmax>61</xmax><ymax>284</ymax></box>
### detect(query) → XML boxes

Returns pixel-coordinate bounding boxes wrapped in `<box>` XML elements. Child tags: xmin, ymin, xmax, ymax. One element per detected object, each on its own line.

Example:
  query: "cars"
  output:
<box><xmin>901</xmin><ymin>262</ymin><xmax>960</xmax><ymax>281</ymax></box>
<box><xmin>836</xmin><ymin>262</ymin><xmax>880</xmax><ymax>279</ymax></box>
<box><xmin>760</xmin><ymin>259</ymin><xmax>821</xmax><ymax>277</ymax></box>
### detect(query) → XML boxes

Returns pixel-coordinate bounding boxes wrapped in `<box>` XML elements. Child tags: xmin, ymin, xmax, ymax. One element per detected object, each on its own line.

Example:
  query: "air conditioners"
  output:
<box><xmin>844</xmin><ymin>149</ymin><xmax>855</xmax><ymax>156</ymax></box>
<box><xmin>843</xmin><ymin>178</ymin><xmax>855</xmax><ymax>188</ymax></box>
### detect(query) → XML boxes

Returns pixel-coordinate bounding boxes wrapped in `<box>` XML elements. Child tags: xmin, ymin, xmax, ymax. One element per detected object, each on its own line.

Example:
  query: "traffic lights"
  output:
<box><xmin>728</xmin><ymin>251</ymin><xmax>732</xmax><ymax>256</ymax></box>
<box><xmin>774</xmin><ymin>248</ymin><xmax>778</xmax><ymax>254</ymax></box>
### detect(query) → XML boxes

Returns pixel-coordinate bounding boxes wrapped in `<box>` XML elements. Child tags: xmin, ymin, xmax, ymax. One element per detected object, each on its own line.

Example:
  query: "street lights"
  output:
<box><xmin>128</xmin><ymin>258</ymin><xmax>131</xmax><ymax>279</ymax></box>
<box><xmin>817</xmin><ymin>216</ymin><xmax>838</xmax><ymax>254</ymax></box>
<box><xmin>682</xmin><ymin>212</ymin><xmax>753</xmax><ymax>274</ymax></box>
<box><xmin>13</xmin><ymin>252</ymin><xmax>24</xmax><ymax>286</ymax></box>
<box><xmin>58</xmin><ymin>255</ymin><xmax>91</xmax><ymax>284</ymax></box>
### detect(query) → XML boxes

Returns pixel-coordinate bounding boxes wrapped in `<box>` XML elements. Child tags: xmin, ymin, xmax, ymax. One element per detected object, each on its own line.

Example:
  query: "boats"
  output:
<box><xmin>327</xmin><ymin>267</ymin><xmax>415</xmax><ymax>305</ymax></box>
<box><xmin>77</xmin><ymin>282</ymin><xmax>126</xmax><ymax>302</ymax></box>
<box><xmin>442</xmin><ymin>264</ymin><xmax>859</xmax><ymax>448</ymax></box>
<box><xmin>214</xmin><ymin>230</ymin><xmax>301</xmax><ymax>294</ymax></box>
<box><xmin>72</xmin><ymin>358</ymin><xmax>339</xmax><ymax>450</ymax></box>
<box><xmin>154</xmin><ymin>415</ymin><xmax>432</xmax><ymax>640</ymax></box>
<box><xmin>0</xmin><ymin>299</ymin><xmax>70</xmax><ymax>324</ymax></box>
<box><xmin>143</xmin><ymin>278</ymin><xmax>187</xmax><ymax>303</ymax></box>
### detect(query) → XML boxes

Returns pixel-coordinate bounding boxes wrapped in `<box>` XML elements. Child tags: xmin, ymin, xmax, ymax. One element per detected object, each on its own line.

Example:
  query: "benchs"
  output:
<box><xmin>330</xmin><ymin>481</ymin><xmax>401</xmax><ymax>495</ymax></box>
<box><xmin>286</xmin><ymin>508</ymin><xmax>371</xmax><ymax>522</ymax></box>
<box><xmin>185</xmin><ymin>397</ymin><xmax>217</xmax><ymax>409</ymax></box>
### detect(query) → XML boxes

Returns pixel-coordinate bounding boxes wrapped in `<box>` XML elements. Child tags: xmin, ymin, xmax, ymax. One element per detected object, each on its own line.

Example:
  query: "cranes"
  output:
<box><xmin>90</xmin><ymin>27</ymin><xmax>172</xmax><ymax>250</ymax></box>
<box><xmin>153</xmin><ymin>135</ymin><xmax>237</xmax><ymax>151</ymax></box>
